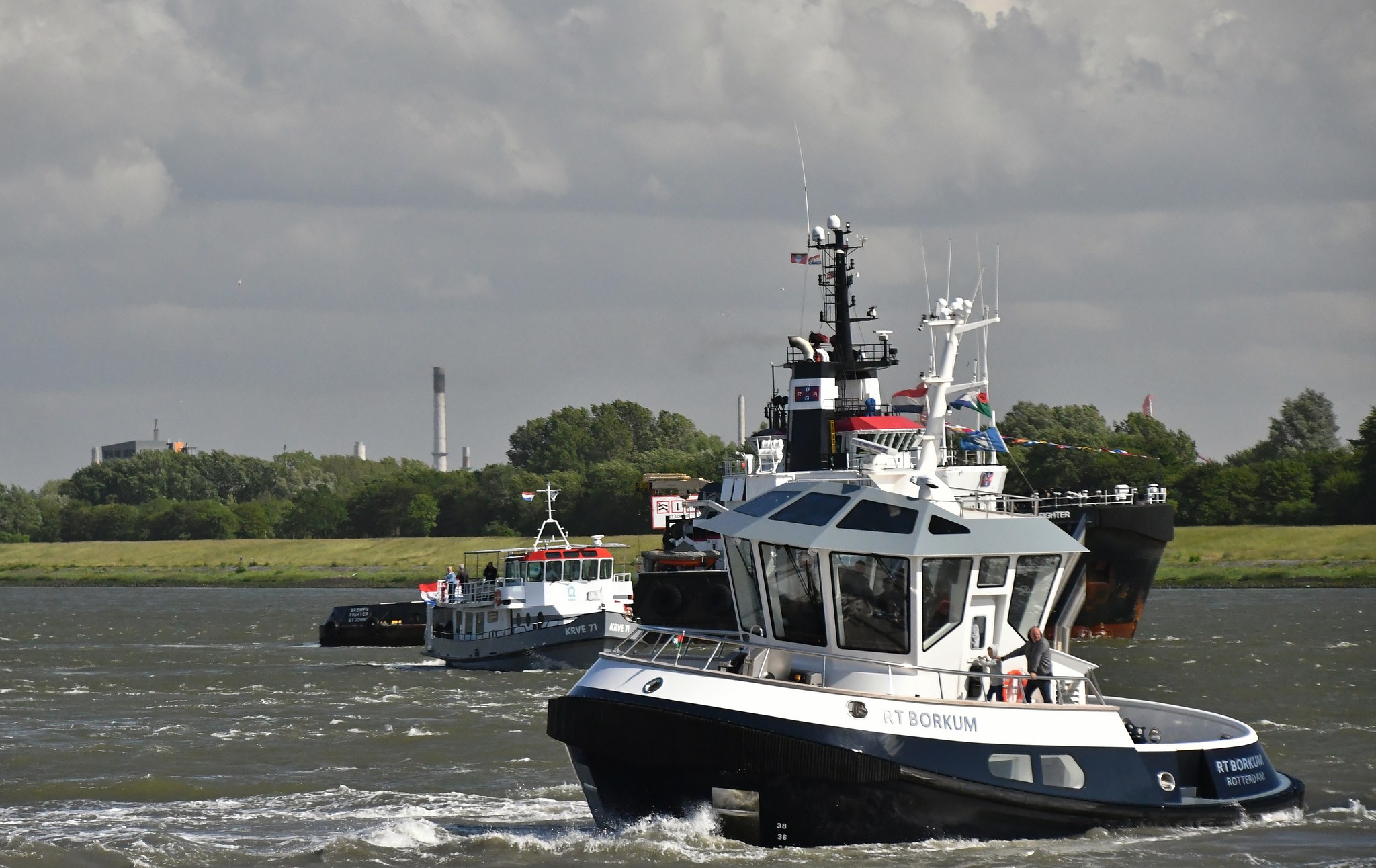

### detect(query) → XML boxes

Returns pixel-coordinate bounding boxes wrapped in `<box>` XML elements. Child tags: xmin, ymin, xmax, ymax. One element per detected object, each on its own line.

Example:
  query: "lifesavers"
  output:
<box><xmin>1002</xmin><ymin>670</ymin><xmax>1028</xmax><ymax>703</ymax></box>
<box><xmin>363</xmin><ymin>616</ymin><xmax>380</xmax><ymax>636</ymax></box>
<box><xmin>494</xmin><ymin>589</ymin><xmax>501</xmax><ymax>606</ymax></box>
<box><xmin>324</xmin><ymin>619</ymin><xmax>339</xmax><ymax>638</ymax></box>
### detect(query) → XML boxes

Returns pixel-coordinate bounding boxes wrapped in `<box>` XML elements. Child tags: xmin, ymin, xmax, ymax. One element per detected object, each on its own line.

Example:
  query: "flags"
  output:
<box><xmin>950</xmin><ymin>391</ymin><xmax>993</xmax><ymax>419</ymax></box>
<box><xmin>545</xmin><ymin>490</ymin><xmax>559</xmax><ymax>499</ymax></box>
<box><xmin>918</xmin><ymin>415</ymin><xmax>1149</xmax><ymax>458</ymax></box>
<box><xmin>891</xmin><ymin>382</ymin><xmax>930</xmax><ymax>414</ymax></box>
<box><xmin>960</xmin><ymin>425</ymin><xmax>1009</xmax><ymax>454</ymax></box>
<box><xmin>808</xmin><ymin>254</ymin><xmax>822</xmax><ymax>265</ymax></box>
<box><xmin>791</xmin><ymin>253</ymin><xmax>808</xmax><ymax>264</ymax></box>
<box><xmin>522</xmin><ymin>491</ymin><xmax>535</xmax><ymax>502</ymax></box>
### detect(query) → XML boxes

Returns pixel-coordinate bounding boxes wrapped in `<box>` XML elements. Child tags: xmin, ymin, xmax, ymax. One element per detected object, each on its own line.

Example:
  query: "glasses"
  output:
<box><xmin>448</xmin><ymin>569</ymin><xmax>452</xmax><ymax>571</ymax></box>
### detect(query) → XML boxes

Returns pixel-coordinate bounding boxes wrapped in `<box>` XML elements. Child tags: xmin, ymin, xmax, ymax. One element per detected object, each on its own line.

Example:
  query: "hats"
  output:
<box><xmin>459</xmin><ymin>564</ymin><xmax>464</xmax><ymax>569</ymax></box>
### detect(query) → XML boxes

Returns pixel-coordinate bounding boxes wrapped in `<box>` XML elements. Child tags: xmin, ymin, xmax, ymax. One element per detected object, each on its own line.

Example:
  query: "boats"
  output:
<box><xmin>318</xmin><ymin>601</ymin><xmax>426</xmax><ymax>647</ymax></box>
<box><xmin>419</xmin><ymin>480</ymin><xmax>648</xmax><ymax>671</ymax></box>
<box><xmin>545</xmin><ymin>114</ymin><xmax>1307</xmax><ymax>851</ymax></box>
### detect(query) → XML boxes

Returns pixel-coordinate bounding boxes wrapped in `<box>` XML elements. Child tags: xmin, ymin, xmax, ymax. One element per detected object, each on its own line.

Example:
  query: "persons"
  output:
<box><xmin>456</xmin><ymin>563</ymin><xmax>469</xmax><ymax>593</ymax></box>
<box><xmin>662</xmin><ymin>524</ymin><xmax>684</xmax><ymax>551</ymax></box>
<box><xmin>484</xmin><ymin>561</ymin><xmax>497</xmax><ymax>584</ymax></box>
<box><xmin>841</xmin><ymin>559</ymin><xmax>952</xmax><ymax>632</ymax></box>
<box><xmin>442</xmin><ymin>564</ymin><xmax>458</xmax><ymax>604</ymax></box>
<box><xmin>639</xmin><ymin>574</ymin><xmax>734</xmax><ymax>628</ymax></box>
<box><xmin>995</xmin><ymin>627</ymin><xmax>1053</xmax><ymax>704</ymax></box>
<box><xmin>978</xmin><ymin>644</ymin><xmax>1004</xmax><ymax>702</ymax></box>
<box><xmin>533</xmin><ymin>560</ymin><xmax>561</xmax><ymax>582</ymax></box>
<box><xmin>864</xmin><ymin>393</ymin><xmax>876</xmax><ymax>417</ymax></box>
